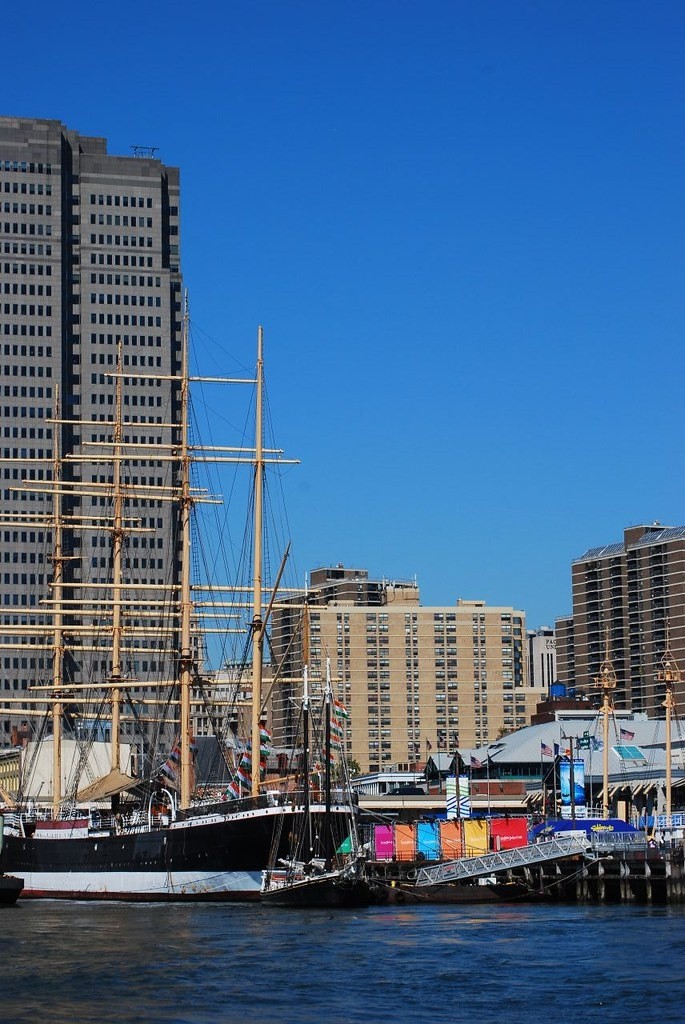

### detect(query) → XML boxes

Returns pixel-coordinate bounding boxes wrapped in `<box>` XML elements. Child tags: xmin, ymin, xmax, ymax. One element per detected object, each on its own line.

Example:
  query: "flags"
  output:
<box><xmin>160</xmin><ymin>736</ymin><xmax>199</xmax><ymax>781</ymax></box>
<box><xmin>541</xmin><ymin>743</ymin><xmax>553</xmax><ymax>756</ymax></box>
<box><xmin>221</xmin><ymin>722</ymin><xmax>272</xmax><ymax>798</ymax></box>
<box><xmin>454</xmin><ymin>733</ymin><xmax>459</xmax><ymax>746</ymax></box>
<box><xmin>439</xmin><ymin>736</ymin><xmax>444</xmax><ymax>747</ymax></box>
<box><xmin>554</xmin><ymin>729</ymin><xmax>604</xmax><ymax>755</ymax></box>
<box><xmin>309</xmin><ymin>699</ymin><xmax>349</xmax><ymax>790</ymax></box>
<box><xmin>488</xmin><ymin>755</ymin><xmax>496</xmax><ymax>771</ymax></box>
<box><xmin>471</xmin><ymin>756</ymin><xmax>482</xmax><ymax>768</ymax></box>
<box><xmin>621</xmin><ymin>729</ymin><xmax>635</xmax><ymax>740</ymax></box>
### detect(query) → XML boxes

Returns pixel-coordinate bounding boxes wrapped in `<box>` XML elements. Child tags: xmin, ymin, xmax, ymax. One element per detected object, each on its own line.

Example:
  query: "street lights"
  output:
<box><xmin>486</xmin><ymin>744</ymin><xmax>506</xmax><ymax>815</ymax></box>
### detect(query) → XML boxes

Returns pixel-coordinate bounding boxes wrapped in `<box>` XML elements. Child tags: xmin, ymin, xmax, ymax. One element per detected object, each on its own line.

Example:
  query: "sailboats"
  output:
<box><xmin>2</xmin><ymin>323</ymin><xmax>389</xmax><ymax>911</ymax></box>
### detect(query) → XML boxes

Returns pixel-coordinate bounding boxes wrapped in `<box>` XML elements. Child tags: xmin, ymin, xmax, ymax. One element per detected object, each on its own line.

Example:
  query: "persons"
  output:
<box><xmin>649</xmin><ymin>839</ymin><xmax>657</xmax><ymax>849</ymax></box>
<box><xmin>660</xmin><ymin>836</ymin><xmax>665</xmax><ymax>854</ymax></box>
